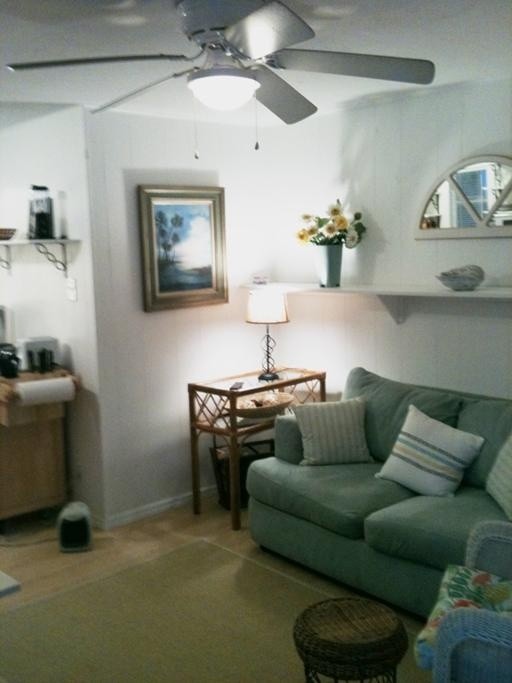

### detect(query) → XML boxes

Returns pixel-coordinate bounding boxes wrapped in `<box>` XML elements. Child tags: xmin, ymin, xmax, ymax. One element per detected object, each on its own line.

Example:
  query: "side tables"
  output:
<box><xmin>188</xmin><ymin>368</ymin><xmax>327</xmax><ymax>531</ymax></box>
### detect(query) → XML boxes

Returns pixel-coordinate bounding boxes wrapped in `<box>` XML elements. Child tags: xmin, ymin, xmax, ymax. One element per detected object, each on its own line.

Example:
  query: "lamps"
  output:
<box><xmin>245</xmin><ymin>273</ymin><xmax>289</xmax><ymax>381</ymax></box>
<box><xmin>188</xmin><ymin>68</ymin><xmax>260</xmax><ymax>160</ymax></box>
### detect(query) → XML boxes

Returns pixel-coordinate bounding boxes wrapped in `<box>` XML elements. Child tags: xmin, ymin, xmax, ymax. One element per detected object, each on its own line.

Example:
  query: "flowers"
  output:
<box><xmin>296</xmin><ymin>206</ymin><xmax>366</xmax><ymax>249</ymax></box>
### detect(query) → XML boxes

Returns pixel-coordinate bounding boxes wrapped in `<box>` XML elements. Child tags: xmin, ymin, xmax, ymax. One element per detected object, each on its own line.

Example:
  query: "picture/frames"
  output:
<box><xmin>137</xmin><ymin>184</ymin><xmax>229</xmax><ymax>313</ymax></box>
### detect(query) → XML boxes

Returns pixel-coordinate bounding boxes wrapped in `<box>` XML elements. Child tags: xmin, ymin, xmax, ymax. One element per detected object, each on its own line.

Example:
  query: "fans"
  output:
<box><xmin>3</xmin><ymin>0</ymin><xmax>435</xmax><ymax>127</ymax></box>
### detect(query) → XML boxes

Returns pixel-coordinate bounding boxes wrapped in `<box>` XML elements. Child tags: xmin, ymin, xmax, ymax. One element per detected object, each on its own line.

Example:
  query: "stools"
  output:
<box><xmin>294</xmin><ymin>597</ymin><xmax>409</xmax><ymax>683</ymax></box>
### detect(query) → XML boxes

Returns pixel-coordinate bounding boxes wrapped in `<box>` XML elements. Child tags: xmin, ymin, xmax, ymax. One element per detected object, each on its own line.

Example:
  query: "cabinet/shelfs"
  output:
<box><xmin>0</xmin><ymin>239</ymin><xmax>80</xmax><ymax>279</ymax></box>
<box><xmin>238</xmin><ymin>283</ymin><xmax>511</xmax><ymax>325</ymax></box>
<box><xmin>0</xmin><ymin>363</ymin><xmax>81</xmax><ymax>528</ymax></box>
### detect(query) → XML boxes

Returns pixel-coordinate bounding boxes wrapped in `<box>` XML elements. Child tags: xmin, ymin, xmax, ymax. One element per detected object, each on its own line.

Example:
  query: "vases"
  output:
<box><xmin>325</xmin><ymin>244</ymin><xmax>342</xmax><ymax>288</ymax></box>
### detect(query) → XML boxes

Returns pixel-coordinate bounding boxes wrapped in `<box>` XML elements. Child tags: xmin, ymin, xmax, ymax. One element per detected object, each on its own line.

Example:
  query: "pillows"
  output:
<box><xmin>291</xmin><ymin>394</ymin><xmax>373</xmax><ymax>466</ymax></box>
<box><xmin>486</xmin><ymin>437</ymin><xmax>512</xmax><ymax>520</ymax></box>
<box><xmin>375</xmin><ymin>404</ymin><xmax>484</xmax><ymax>498</ymax></box>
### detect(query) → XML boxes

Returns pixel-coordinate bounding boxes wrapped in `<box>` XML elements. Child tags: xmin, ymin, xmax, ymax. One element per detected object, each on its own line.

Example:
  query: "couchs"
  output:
<box><xmin>245</xmin><ymin>366</ymin><xmax>512</xmax><ymax>619</ymax></box>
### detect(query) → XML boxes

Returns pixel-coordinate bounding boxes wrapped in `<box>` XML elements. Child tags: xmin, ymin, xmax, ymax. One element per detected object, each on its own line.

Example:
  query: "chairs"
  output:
<box><xmin>412</xmin><ymin>520</ymin><xmax>512</xmax><ymax>683</ymax></box>
